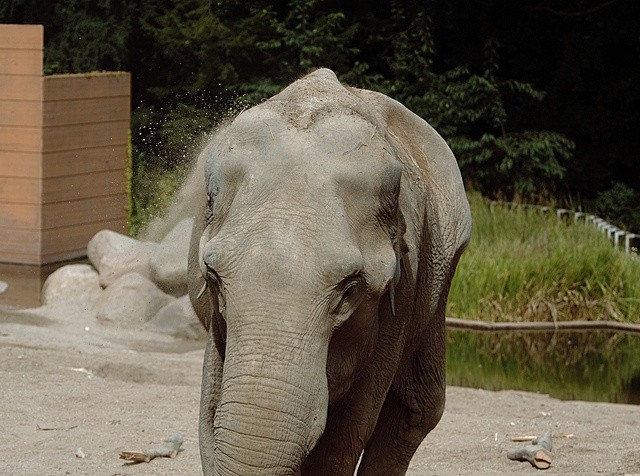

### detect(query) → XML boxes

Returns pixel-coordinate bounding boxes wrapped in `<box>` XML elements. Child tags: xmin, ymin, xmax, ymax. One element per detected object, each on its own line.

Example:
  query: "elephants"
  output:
<box><xmin>185</xmin><ymin>68</ymin><xmax>473</xmax><ymax>476</ymax></box>
